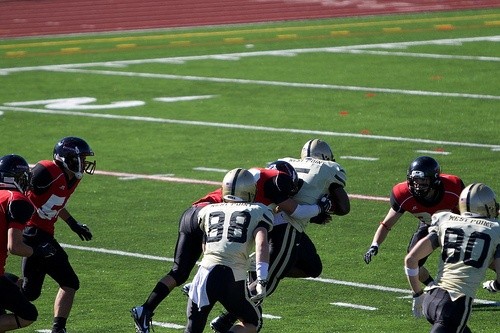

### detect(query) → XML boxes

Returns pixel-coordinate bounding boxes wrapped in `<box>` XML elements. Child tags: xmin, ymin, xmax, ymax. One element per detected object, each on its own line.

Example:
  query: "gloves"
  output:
<box><xmin>482</xmin><ymin>279</ymin><xmax>500</xmax><ymax>294</ymax></box>
<box><xmin>317</xmin><ymin>194</ymin><xmax>334</xmax><ymax>214</ymax></box>
<box><xmin>248</xmin><ymin>276</ymin><xmax>267</xmax><ymax>308</ymax></box>
<box><xmin>309</xmin><ymin>213</ymin><xmax>333</xmax><ymax>225</ymax></box>
<box><xmin>66</xmin><ymin>217</ymin><xmax>92</xmax><ymax>241</ymax></box>
<box><xmin>364</xmin><ymin>245</ymin><xmax>379</xmax><ymax>265</ymax></box>
<box><xmin>29</xmin><ymin>242</ymin><xmax>57</xmax><ymax>259</ymax></box>
<box><xmin>411</xmin><ymin>289</ymin><xmax>425</xmax><ymax>317</ymax></box>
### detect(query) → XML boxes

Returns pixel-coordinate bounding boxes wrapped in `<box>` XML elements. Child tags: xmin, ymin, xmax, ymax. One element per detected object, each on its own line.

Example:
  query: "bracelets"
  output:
<box><xmin>380</xmin><ymin>221</ymin><xmax>392</xmax><ymax>232</ymax></box>
<box><xmin>404</xmin><ymin>266</ymin><xmax>420</xmax><ymax>277</ymax></box>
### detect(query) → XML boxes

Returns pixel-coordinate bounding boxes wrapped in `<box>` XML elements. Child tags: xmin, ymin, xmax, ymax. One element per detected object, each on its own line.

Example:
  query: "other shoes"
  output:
<box><xmin>51</xmin><ymin>327</ymin><xmax>66</xmax><ymax>333</ymax></box>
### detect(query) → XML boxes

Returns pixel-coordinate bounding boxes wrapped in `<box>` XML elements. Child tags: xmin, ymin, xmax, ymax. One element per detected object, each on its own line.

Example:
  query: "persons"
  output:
<box><xmin>404</xmin><ymin>183</ymin><xmax>500</xmax><ymax>333</ymax></box>
<box><xmin>210</xmin><ymin>138</ymin><xmax>350</xmax><ymax>333</ymax></box>
<box><xmin>363</xmin><ymin>156</ymin><xmax>466</xmax><ymax>286</ymax></box>
<box><xmin>20</xmin><ymin>137</ymin><xmax>97</xmax><ymax>333</ymax></box>
<box><xmin>130</xmin><ymin>159</ymin><xmax>332</xmax><ymax>333</ymax></box>
<box><xmin>185</xmin><ymin>167</ymin><xmax>274</xmax><ymax>333</ymax></box>
<box><xmin>0</xmin><ymin>154</ymin><xmax>54</xmax><ymax>333</ymax></box>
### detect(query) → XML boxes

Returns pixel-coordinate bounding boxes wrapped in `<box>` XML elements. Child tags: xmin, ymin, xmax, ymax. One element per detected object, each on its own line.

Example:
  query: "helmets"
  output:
<box><xmin>264</xmin><ymin>160</ymin><xmax>299</xmax><ymax>196</ymax></box>
<box><xmin>52</xmin><ymin>137</ymin><xmax>97</xmax><ymax>178</ymax></box>
<box><xmin>221</xmin><ymin>168</ymin><xmax>256</xmax><ymax>203</ymax></box>
<box><xmin>300</xmin><ymin>139</ymin><xmax>336</xmax><ymax>164</ymax></box>
<box><xmin>0</xmin><ymin>155</ymin><xmax>33</xmax><ymax>197</ymax></box>
<box><xmin>405</xmin><ymin>155</ymin><xmax>445</xmax><ymax>207</ymax></box>
<box><xmin>459</xmin><ymin>182</ymin><xmax>500</xmax><ymax>221</ymax></box>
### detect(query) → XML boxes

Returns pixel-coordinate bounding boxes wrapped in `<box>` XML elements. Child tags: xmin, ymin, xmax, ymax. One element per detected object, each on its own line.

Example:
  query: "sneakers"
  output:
<box><xmin>181</xmin><ymin>283</ymin><xmax>193</xmax><ymax>297</ymax></box>
<box><xmin>129</xmin><ymin>306</ymin><xmax>155</xmax><ymax>333</ymax></box>
<box><xmin>208</xmin><ymin>314</ymin><xmax>233</xmax><ymax>333</ymax></box>
